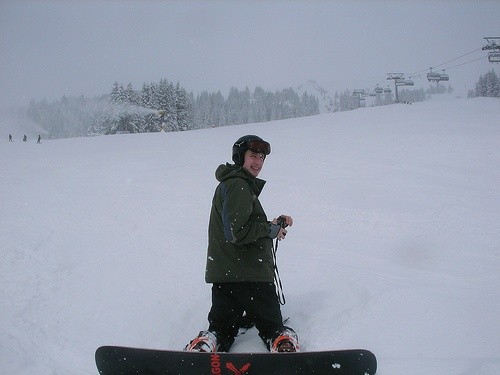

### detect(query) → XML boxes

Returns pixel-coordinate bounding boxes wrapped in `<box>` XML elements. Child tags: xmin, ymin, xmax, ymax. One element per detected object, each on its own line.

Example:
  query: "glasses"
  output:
<box><xmin>246</xmin><ymin>140</ymin><xmax>270</xmax><ymax>155</ymax></box>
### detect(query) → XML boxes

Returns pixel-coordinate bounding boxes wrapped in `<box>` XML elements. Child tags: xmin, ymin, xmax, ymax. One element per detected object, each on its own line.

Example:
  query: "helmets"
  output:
<box><xmin>231</xmin><ymin>135</ymin><xmax>271</xmax><ymax>165</ymax></box>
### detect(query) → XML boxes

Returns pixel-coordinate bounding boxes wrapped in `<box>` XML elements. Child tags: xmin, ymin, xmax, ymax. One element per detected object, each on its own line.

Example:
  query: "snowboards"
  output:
<box><xmin>95</xmin><ymin>344</ymin><xmax>377</xmax><ymax>375</ymax></box>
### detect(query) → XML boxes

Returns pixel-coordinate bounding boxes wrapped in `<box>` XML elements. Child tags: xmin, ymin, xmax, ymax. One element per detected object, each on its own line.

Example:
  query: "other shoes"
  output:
<box><xmin>190</xmin><ymin>331</ymin><xmax>217</xmax><ymax>352</ymax></box>
<box><xmin>276</xmin><ymin>340</ymin><xmax>295</xmax><ymax>352</ymax></box>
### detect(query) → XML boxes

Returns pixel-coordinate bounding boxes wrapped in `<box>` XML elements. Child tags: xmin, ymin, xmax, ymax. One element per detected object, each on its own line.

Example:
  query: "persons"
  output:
<box><xmin>8</xmin><ymin>133</ymin><xmax>13</xmax><ymax>142</ymax></box>
<box><xmin>22</xmin><ymin>134</ymin><xmax>27</xmax><ymax>141</ymax></box>
<box><xmin>183</xmin><ymin>135</ymin><xmax>300</xmax><ymax>354</ymax></box>
<box><xmin>401</xmin><ymin>99</ymin><xmax>412</xmax><ymax>105</ymax></box>
<box><xmin>37</xmin><ymin>134</ymin><xmax>41</xmax><ymax>144</ymax></box>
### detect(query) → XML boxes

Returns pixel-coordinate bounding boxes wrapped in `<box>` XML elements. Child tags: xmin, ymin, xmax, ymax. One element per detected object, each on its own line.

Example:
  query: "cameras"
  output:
<box><xmin>277</xmin><ymin>216</ymin><xmax>288</xmax><ymax>236</ymax></box>
